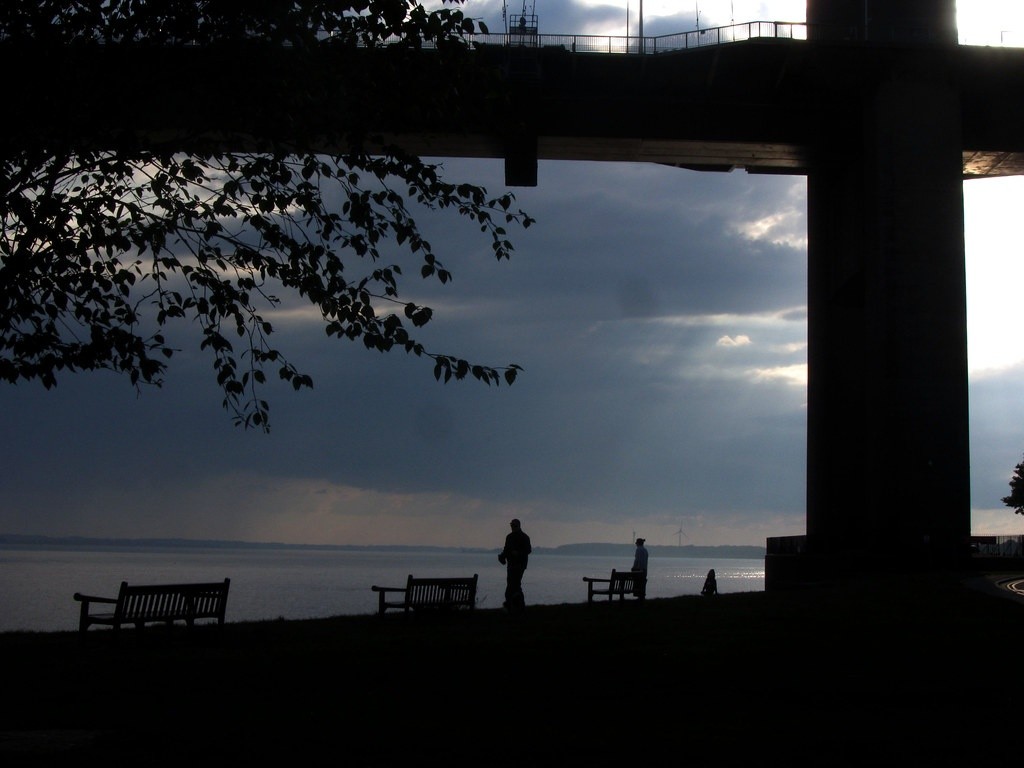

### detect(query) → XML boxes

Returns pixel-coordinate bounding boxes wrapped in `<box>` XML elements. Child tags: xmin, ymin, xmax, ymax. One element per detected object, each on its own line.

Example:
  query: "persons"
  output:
<box><xmin>700</xmin><ymin>569</ymin><xmax>718</xmax><ymax>595</ymax></box>
<box><xmin>632</xmin><ymin>537</ymin><xmax>648</xmax><ymax>599</ymax></box>
<box><xmin>497</xmin><ymin>519</ymin><xmax>531</xmax><ymax>606</ymax></box>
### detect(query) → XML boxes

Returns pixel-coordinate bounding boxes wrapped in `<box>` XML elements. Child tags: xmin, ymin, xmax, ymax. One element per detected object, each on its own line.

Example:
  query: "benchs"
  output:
<box><xmin>371</xmin><ymin>574</ymin><xmax>478</xmax><ymax>617</ymax></box>
<box><xmin>73</xmin><ymin>577</ymin><xmax>232</xmax><ymax>632</ymax></box>
<box><xmin>582</xmin><ymin>569</ymin><xmax>648</xmax><ymax>603</ymax></box>
